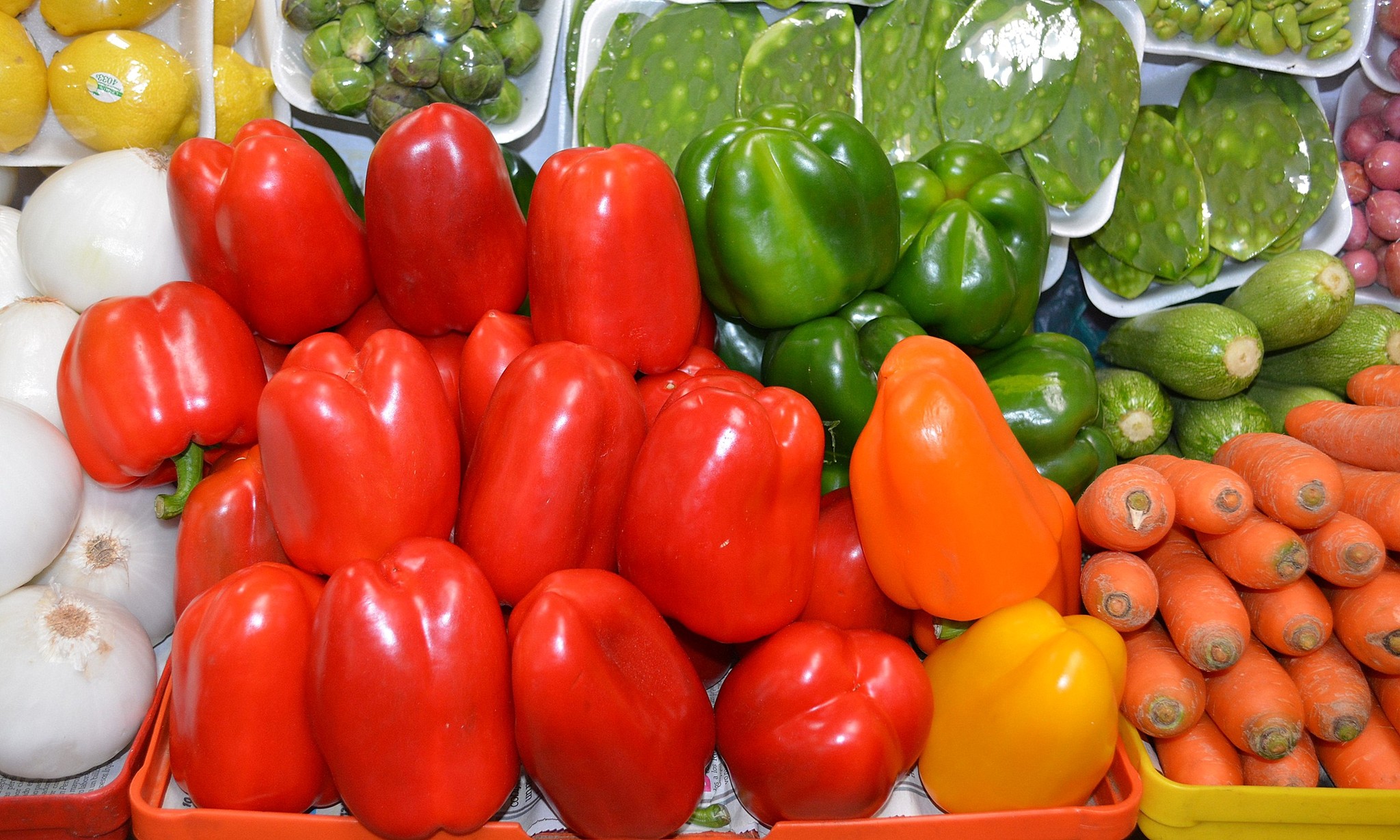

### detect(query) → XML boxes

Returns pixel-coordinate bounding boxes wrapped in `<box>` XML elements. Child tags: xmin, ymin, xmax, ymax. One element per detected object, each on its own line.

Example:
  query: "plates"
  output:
<box><xmin>2</xmin><ymin>1</ymin><xmax>1400</xmax><ymax>315</ymax></box>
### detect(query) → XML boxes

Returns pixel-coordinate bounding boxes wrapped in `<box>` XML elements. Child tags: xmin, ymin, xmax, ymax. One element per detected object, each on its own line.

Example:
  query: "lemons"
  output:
<box><xmin>0</xmin><ymin>0</ymin><xmax>277</xmax><ymax>160</ymax></box>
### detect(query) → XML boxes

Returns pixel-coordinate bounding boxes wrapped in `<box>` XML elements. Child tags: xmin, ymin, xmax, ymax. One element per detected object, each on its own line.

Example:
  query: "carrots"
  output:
<box><xmin>1075</xmin><ymin>362</ymin><xmax>1400</xmax><ymax>788</ymax></box>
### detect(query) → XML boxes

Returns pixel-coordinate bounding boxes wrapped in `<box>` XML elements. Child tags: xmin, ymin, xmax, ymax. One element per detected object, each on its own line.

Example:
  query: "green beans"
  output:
<box><xmin>1134</xmin><ymin>0</ymin><xmax>1355</xmax><ymax>59</ymax></box>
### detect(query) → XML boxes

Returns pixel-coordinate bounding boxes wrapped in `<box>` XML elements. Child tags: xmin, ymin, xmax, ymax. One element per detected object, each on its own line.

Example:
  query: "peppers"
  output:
<box><xmin>55</xmin><ymin>104</ymin><xmax>1129</xmax><ymax>840</ymax></box>
